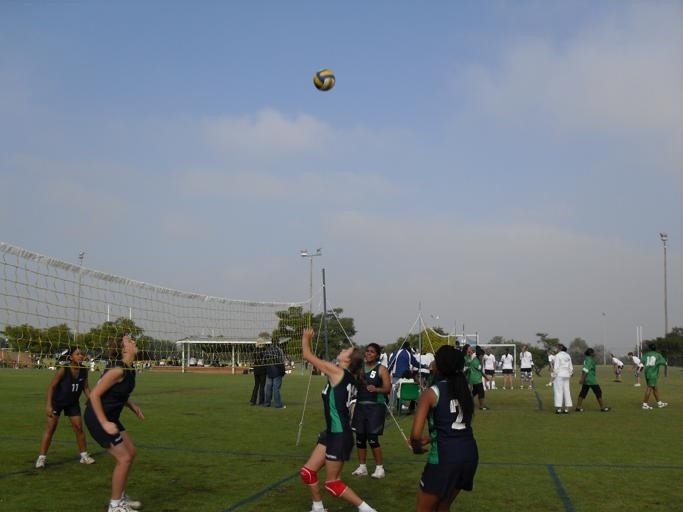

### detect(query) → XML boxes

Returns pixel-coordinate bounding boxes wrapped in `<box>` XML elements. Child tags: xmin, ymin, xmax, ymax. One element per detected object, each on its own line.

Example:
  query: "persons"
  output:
<box><xmin>84</xmin><ymin>333</ymin><xmax>145</xmax><ymax>512</ymax></box>
<box><xmin>35</xmin><ymin>345</ymin><xmax>96</xmax><ymax>467</ymax></box>
<box><xmin>262</xmin><ymin>337</ymin><xmax>287</xmax><ymax>408</ymax></box>
<box><xmin>300</xmin><ymin>327</ymin><xmax>377</xmax><ymax>512</ymax></box>
<box><xmin>250</xmin><ymin>338</ymin><xmax>267</xmax><ymax>405</ymax></box>
<box><xmin>352</xmin><ymin>342</ymin><xmax>390</xmax><ymax>480</ymax></box>
<box><xmin>639</xmin><ymin>343</ymin><xmax>669</xmax><ymax>410</ymax></box>
<box><xmin>378</xmin><ymin>341</ymin><xmax>645</xmax><ymax>414</ymax></box>
<box><xmin>410</xmin><ymin>345</ymin><xmax>478</xmax><ymax>512</ymax></box>
<box><xmin>0</xmin><ymin>339</ymin><xmax>296</xmax><ymax>374</ymax></box>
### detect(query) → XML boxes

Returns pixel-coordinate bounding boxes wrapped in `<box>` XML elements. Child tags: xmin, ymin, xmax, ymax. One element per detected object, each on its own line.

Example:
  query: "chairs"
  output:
<box><xmin>397</xmin><ymin>383</ymin><xmax>419</xmax><ymax>417</ymax></box>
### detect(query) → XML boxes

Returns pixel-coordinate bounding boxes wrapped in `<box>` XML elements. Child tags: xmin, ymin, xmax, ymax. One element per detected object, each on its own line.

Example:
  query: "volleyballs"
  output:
<box><xmin>315</xmin><ymin>68</ymin><xmax>335</xmax><ymax>91</ymax></box>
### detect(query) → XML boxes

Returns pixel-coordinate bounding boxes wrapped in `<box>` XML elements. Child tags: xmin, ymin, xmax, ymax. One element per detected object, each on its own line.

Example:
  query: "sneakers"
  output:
<box><xmin>108</xmin><ymin>498</ymin><xmax>141</xmax><ymax>512</ymax></box>
<box><xmin>601</xmin><ymin>407</ymin><xmax>610</xmax><ymax>412</ymax></box>
<box><xmin>642</xmin><ymin>403</ymin><xmax>668</xmax><ymax>410</ymax></box>
<box><xmin>371</xmin><ymin>468</ymin><xmax>385</xmax><ymax>479</ymax></box>
<box><xmin>555</xmin><ymin>408</ymin><xmax>584</xmax><ymax>414</ymax></box>
<box><xmin>80</xmin><ymin>457</ymin><xmax>95</xmax><ymax>464</ymax></box>
<box><xmin>352</xmin><ymin>467</ymin><xmax>368</xmax><ymax>476</ymax></box>
<box><xmin>36</xmin><ymin>458</ymin><xmax>46</xmax><ymax>468</ymax></box>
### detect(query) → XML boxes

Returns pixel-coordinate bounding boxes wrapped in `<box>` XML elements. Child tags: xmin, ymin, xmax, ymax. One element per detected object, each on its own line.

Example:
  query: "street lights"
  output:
<box><xmin>659</xmin><ymin>231</ymin><xmax>668</xmax><ymax>338</ymax></box>
<box><xmin>429</xmin><ymin>312</ymin><xmax>440</xmax><ymax>332</ymax></box>
<box><xmin>73</xmin><ymin>250</ymin><xmax>85</xmax><ymax>345</ymax></box>
<box><xmin>299</xmin><ymin>246</ymin><xmax>322</xmax><ymax>330</ymax></box>
